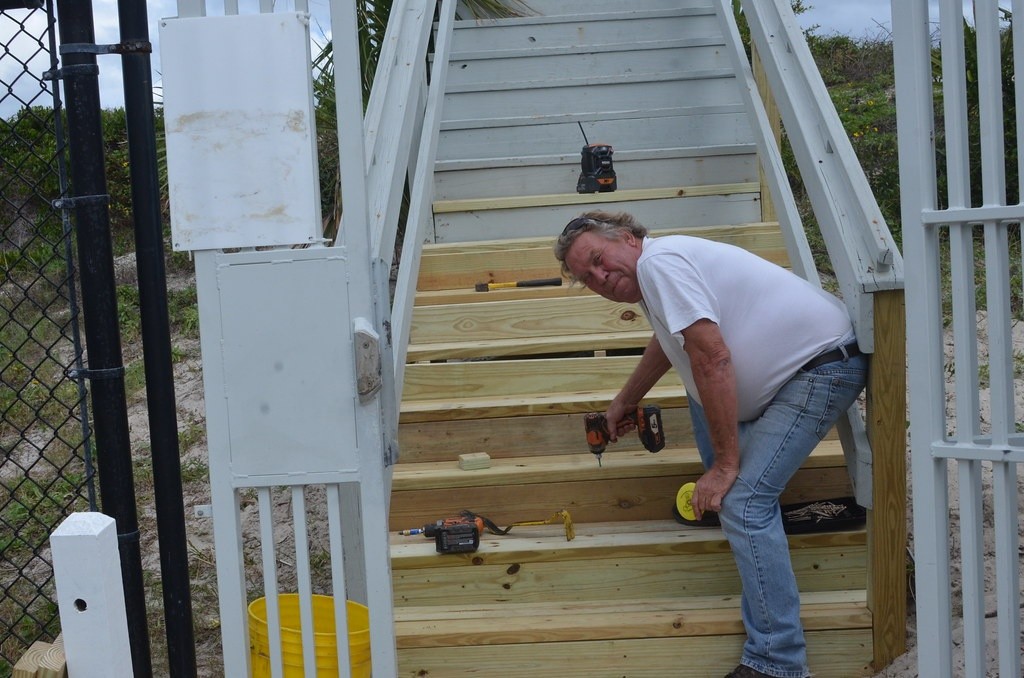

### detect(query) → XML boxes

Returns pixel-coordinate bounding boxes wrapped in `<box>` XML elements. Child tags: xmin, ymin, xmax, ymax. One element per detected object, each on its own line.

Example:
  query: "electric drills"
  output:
<box><xmin>583</xmin><ymin>403</ymin><xmax>667</xmax><ymax>468</ymax></box>
<box><xmin>397</xmin><ymin>511</ymin><xmax>485</xmax><ymax>555</ymax></box>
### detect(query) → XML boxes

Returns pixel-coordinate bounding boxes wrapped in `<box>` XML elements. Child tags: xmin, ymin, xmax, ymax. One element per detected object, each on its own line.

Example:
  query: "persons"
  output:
<box><xmin>557</xmin><ymin>211</ymin><xmax>868</xmax><ymax>678</ymax></box>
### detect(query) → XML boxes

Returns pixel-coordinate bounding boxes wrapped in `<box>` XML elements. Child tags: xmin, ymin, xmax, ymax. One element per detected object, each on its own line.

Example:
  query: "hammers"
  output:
<box><xmin>474</xmin><ymin>277</ymin><xmax>562</xmax><ymax>293</ymax></box>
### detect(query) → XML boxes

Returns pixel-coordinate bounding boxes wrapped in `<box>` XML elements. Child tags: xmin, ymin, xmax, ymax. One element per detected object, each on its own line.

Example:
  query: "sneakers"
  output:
<box><xmin>723</xmin><ymin>664</ymin><xmax>768</xmax><ymax>678</ymax></box>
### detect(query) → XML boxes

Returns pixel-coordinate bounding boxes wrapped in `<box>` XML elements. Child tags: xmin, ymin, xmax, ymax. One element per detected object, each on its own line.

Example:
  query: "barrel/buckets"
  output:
<box><xmin>244</xmin><ymin>592</ymin><xmax>371</xmax><ymax>678</ymax></box>
